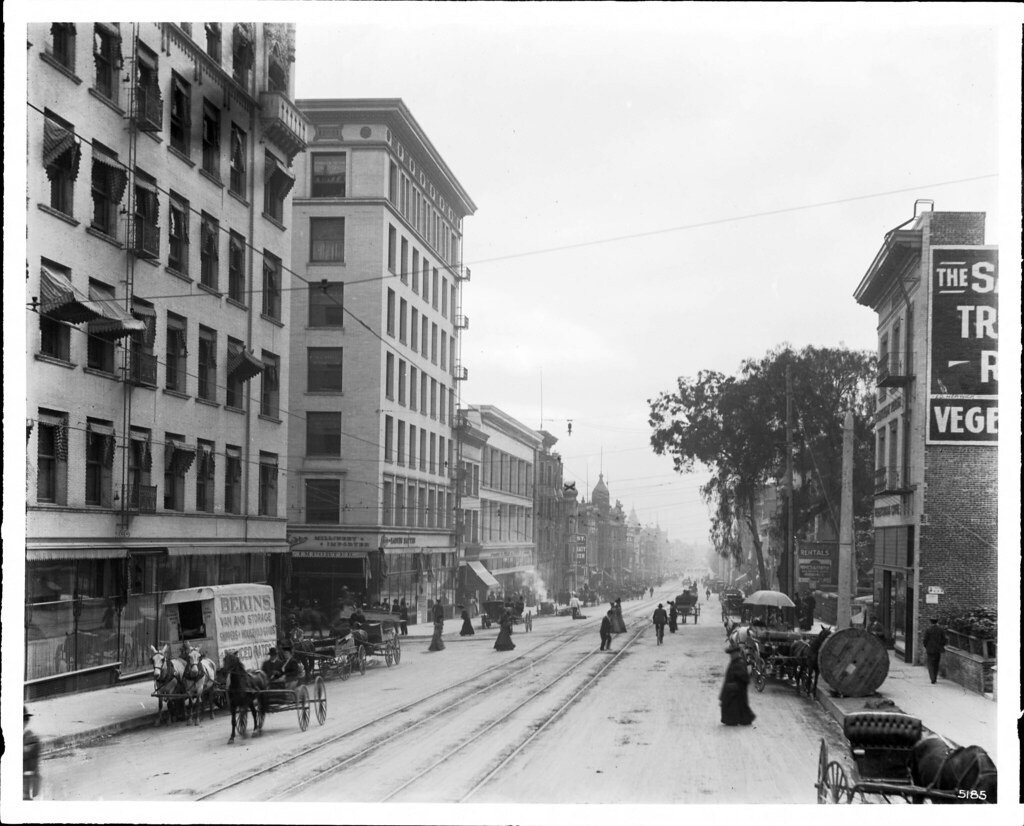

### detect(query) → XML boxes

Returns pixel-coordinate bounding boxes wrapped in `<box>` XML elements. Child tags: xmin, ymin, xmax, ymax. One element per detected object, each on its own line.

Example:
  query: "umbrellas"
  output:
<box><xmin>742</xmin><ymin>590</ymin><xmax>797</xmax><ymax>627</ymax></box>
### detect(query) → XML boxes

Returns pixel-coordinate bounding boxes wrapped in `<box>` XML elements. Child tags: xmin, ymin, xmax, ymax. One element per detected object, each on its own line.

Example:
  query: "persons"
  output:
<box><xmin>262</xmin><ymin>648</ymin><xmax>285</xmax><ymax>682</ymax></box>
<box><xmin>580</xmin><ymin>570</ymin><xmax>745</xmax><ymax>634</ymax></box>
<box><xmin>279</xmin><ymin>586</ymin><xmax>581</xmax><ymax>655</ymax></box>
<box><xmin>279</xmin><ymin>647</ymin><xmax>301</xmax><ymax>691</ymax></box>
<box><xmin>923</xmin><ymin>615</ymin><xmax>947</xmax><ymax>683</ymax></box>
<box><xmin>865</xmin><ymin>612</ymin><xmax>885</xmax><ymax>640</ymax></box>
<box><xmin>718</xmin><ymin>643</ymin><xmax>757</xmax><ymax>727</ymax></box>
<box><xmin>400</xmin><ymin>591</ymin><xmax>450</xmax><ymax>609</ymax></box>
<box><xmin>24</xmin><ymin>705</ymin><xmax>42</xmax><ymax>800</ymax></box>
<box><xmin>793</xmin><ymin>588</ymin><xmax>816</xmax><ymax>631</ymax></box>
<box><xmin>652</xmin><ymin>603</ymin><xmax>669</xmax><ymax>643</ymax></box>
<box><xmin>599</xmin><ymin>610</ymin><xmax>614</xmax><ymax>652</ymax></box>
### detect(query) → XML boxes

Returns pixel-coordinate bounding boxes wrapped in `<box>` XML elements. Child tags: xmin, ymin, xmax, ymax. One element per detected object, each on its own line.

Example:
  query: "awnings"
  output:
<box><xmin>464</xmin><ymin>560</ymin><xmax>502</xmax><ymax>591</ymax></box>
<box><xmin>39</xmin><ymin>265</ymin><xmax>266</xmax><ymax>386</ymax></box>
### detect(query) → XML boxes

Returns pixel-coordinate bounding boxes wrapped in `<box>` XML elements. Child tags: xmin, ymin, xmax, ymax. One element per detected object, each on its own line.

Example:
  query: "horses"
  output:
<box><xmin>149</xmin><ymin>623</ymin><xmax>370</xmax><ymax>745</ymax></box>
<box><xmin>909</xmin><ymin>734</ymin><xmax>997</xmax><ymax>804</ymax></box>
<box><xmin>790</xmin><ymin>624</ymin><xmax>831</xmax><ymax>700</ymax></box>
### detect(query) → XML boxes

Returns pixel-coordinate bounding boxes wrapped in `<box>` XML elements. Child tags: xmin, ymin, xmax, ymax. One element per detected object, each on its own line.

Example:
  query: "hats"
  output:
<box><xmin>615</xmin><ymin>598</ymin><xmax>621</xmax><ymax>603</ymax></box>
<box><xmin>725</xmin><ymin>646</ymin><xmax>740</xmax><ymax>654</ymax></box>
<box><xmin>667</xmin><ymin>601</ymin><xmax>674</xmax><ymax>605</ymax></box>
<box><xmin>930</xmin><ymin>618</ymin><xmax>938</xmax><ymax>624</ymax></box>
<box><xmin>268</xmin><ymin>647</ymin><xmax>278</xmax><ymax>655</ymax></box>
<box><xmin>23</xmin><ymin>706</ymin><xmax>34</xmax><ymax>717</ymax></box>
<box><xmin>658</xmin><ymin>604</ymin><xmax>664</xmax><ymax>607</ymax></box>
<box><xmin>282</xmin><ymin>646</ymin><xmax>293</xmax><ymax>651</ymax></box>
<box><xmin>289</xmin><ymin>614</ymin><xmax>295</xmax><ymax>619</ymax></box>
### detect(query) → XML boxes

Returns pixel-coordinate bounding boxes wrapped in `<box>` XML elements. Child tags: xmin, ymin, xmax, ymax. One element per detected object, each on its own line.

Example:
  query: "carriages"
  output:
<box><xmin>220</xmin><ymin>647</ymin><xmax>330</xmax><ymax>747</ymax></box>
<box><xmin>813</xmin><ymin>698</ymin><xmax>996</xmax><ymax>805</ymax></box>
<box><xmin>285</xmin><ymin>610</ymin><xmax>407</xmax><ymax>681</ymax></box>
<box><xmin>717</xmin><ymin>583</ymin><xmax>834</xmax><ymax>698</ymax></box>
<box><xmin>147</xmin><ymin>582</ymin><xmax>279</xmax><ymax>728</ymax></box>
